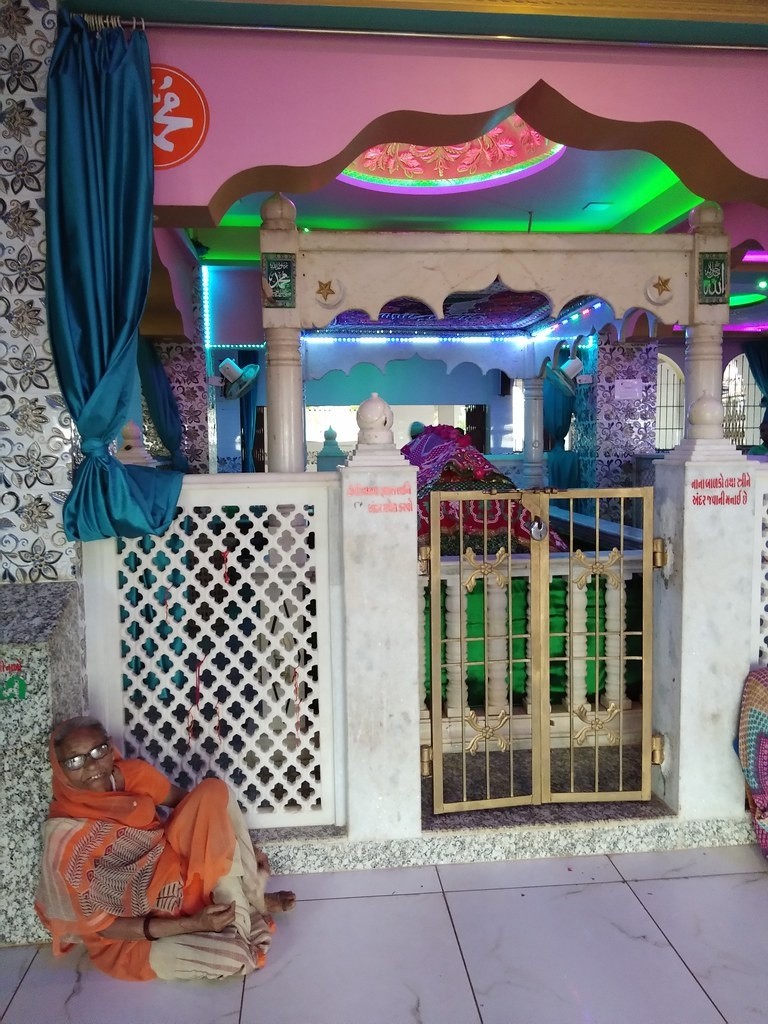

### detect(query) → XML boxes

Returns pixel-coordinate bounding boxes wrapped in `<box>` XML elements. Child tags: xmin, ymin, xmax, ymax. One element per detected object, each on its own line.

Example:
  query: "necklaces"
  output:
<box><xmin>109</xmin><ymin>774</ymin><xmax>118</xmax><ymax>792</ymax></box>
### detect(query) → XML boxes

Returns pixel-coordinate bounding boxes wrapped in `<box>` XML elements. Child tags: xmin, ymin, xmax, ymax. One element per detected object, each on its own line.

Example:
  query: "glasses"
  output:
<box><xmin>59</xmin><ymin>740</ymin><xmax>112</xmax><ymax>771</ymax></box>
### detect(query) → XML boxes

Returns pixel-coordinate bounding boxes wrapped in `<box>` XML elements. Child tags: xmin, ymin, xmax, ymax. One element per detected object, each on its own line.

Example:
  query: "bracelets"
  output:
<box><xmin>143</xmin><ymin>915</ymin><xmax>160</xmax><ymax>941</ymax></box>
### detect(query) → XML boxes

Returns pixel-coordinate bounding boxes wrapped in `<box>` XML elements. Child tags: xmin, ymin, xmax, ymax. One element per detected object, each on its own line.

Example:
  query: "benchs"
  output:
<box><xmin>549</xmin><ymin>505</ymin><xmax>643</xmax><ymax>550</ymax></box>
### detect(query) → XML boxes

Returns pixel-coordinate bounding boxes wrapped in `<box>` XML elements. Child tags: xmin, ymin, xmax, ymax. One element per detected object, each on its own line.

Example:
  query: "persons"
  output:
<box><xmin>410</xmin><ymin>421</ymin><xmax>427</xmax><ymax>440</ymax></box>
<box><xmin>454</xmin><ymin>427</ymin><xmax>464</xmax><ymax>436</ymax></box>
<box><xmin>747</xmin><ymin>422</ymin><xmax>768</xmax><ymax>456</ymax></box>
<box><xmin>32</xmin><ymin>715</ymin><xmax>297</xmax><ymax>983</ymax></box>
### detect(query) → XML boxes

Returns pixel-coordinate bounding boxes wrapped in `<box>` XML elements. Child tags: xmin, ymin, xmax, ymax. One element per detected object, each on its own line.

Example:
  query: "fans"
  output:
<box><xmin>209</xmin><ymin>357</ymin><xmax>261</xmax><ymax>400</ymax></box>
<box><xmin>545</xmin><ymin>355</ymin><xmax>583</xmax><ymax>397</ymax></box>
